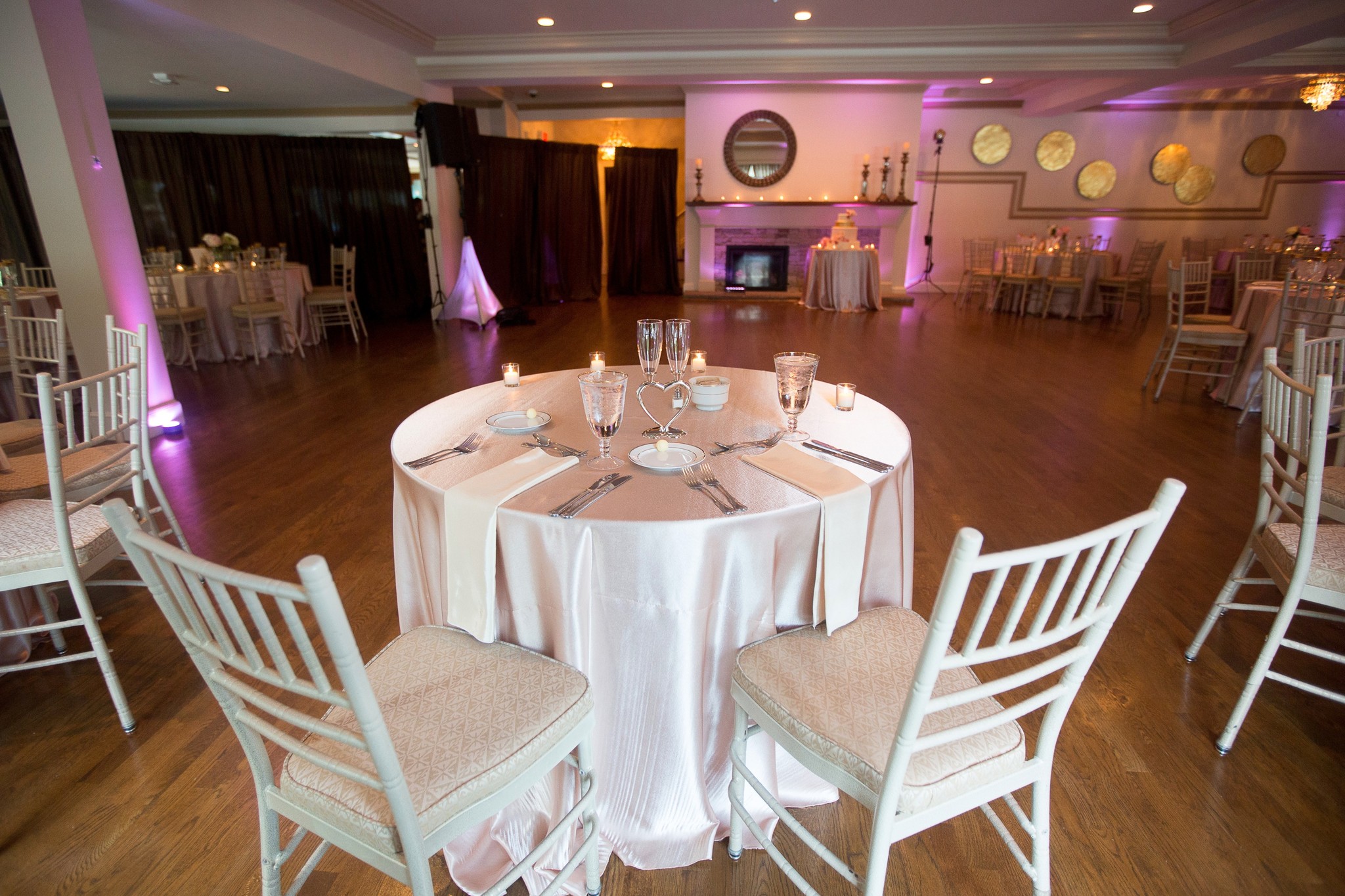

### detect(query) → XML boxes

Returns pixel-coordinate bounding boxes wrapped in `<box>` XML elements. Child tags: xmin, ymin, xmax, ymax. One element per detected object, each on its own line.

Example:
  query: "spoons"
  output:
<box><xmin>532</xmin><ymin>432</ymin><xmax>588</xmax><ymax>457</ymax></box>
<box><xmin>713</xmin><ymin>439</ymin><xmax>772</xmax><ymax>449</ymax></box>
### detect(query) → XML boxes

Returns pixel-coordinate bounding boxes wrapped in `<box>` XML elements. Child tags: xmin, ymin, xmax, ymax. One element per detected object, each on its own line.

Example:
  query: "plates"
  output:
<box><xmin>486</xmin><ymin>410</ymin><xmax>552</xmax><ymax>432</ymax></box>
<box><xmin>628</xmin><ymin>441</ymin><xmax>706</xmax><ymax>471</ymax></box>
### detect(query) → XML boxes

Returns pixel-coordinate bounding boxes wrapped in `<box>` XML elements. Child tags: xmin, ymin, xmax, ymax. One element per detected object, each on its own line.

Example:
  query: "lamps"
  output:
<box><xmin>1298</xmin><ymin>71</ymin><xmax>1345</xmax><ymax>112</ymax></box>
<box><xmin>599</xmin><ymin>119</ymin><xmax>632</xmax><ymax>160</ymax></box>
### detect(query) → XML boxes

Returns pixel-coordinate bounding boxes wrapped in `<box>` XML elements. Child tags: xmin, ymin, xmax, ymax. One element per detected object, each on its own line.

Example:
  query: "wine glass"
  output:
<box><xmin>578</xmin><ymin>371</ymin><xmax>629</xmax><ymax>470</ymax></box>
<box><xmin>774</xmin><ymin>352</ymin><xmax>821</xmax><ymax>441</ymax></box>
<box><xmin>1</xmin><ymin>257</ymin><xmax>22</xmax><ymax>292</ymax></box>
<box><xmin>1287</xmin><ymin>258</ymin><xmax>1345</xmax><ymax>296</ymax></box>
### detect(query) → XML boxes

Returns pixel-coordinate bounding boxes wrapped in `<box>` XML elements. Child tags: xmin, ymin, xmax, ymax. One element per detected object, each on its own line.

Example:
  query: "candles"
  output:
<box><xmin>690</xmin><ymin>350</ymin><xmax>707</xmax><ymax>373</ymax></box>
<box><xmin>883</xmin><ymin>146</ymin><xmax>890</xmax><ymax>157</ymax></box>
<box><xmin>835</xmin><ymin>383</ymin><xmax>856</xmax><ymax>411</ymax></box>
<box><xmin>501</xmin><ymin>363</ymin><xmax>520</xmax><ymax>387</ymax></box>
<box><xmin>863</xmin><ymin>153</ymin><xmax>869</xmax><ymax>164</ymax></box>
<box><xmin>695</xmin><ymin>158</ymin><xmax>702</xmax><ymax>170</ymax></box>
<box><xmin>902</xmin><ymin>142</ymin><xmax>910</xmax><ymax>152</ymax></box>
<box><xmin>588</xmin><ymin>351</ymin><xmax>606</xmax><ymax>371</ymax></box>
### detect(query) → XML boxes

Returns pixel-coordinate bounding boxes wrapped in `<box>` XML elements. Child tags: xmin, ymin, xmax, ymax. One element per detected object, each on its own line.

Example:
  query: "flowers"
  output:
<box><xmin>203</xmin><ymin>233</ymin><xmax>238</xmax><ymax>262</ymax></box>
<box><xmin>1047</xmin><ymin>224</ymin><xmax>1070</xmax><ymax>249</ymax></box>
<box><xmin>1285</xmin><ymin>221</ymin><xmax>1317</xmax><ymax>250</ymax></box>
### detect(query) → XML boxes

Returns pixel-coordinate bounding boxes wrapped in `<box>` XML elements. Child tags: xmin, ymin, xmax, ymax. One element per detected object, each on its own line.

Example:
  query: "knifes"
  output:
<box><xmin>811</xmin><ymin>439</ymin><xmax>894</xmax><ymax>470</ymax></box>
<box><xmin>802</xmin><ymin>442</ymin><xmax>888</xmax><ymax>473</ymax></box>
<box><xmin>561</xmin><ymin>475</ymin><xmax>634</xmax><ymax>519</ymax></box>
<box><xmin>548</xmin><ymin>472</ymin><xmax>620</xmax><ymax>517</ymax></box>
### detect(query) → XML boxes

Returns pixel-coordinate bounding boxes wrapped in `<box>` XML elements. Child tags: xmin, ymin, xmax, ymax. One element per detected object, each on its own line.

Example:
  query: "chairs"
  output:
<box><xmin>1</xmin><ymin>310</ymin><xmax>203</xmax><ymax>652</ymax></box>
<box><xmin>0</xmin><ymin>242</ymin><xmax>367</xmax><ymax>405</ymax></box>
<box><xmin>1219</xmin><ymin>328</ymin><xmax>1345</xmax><ymax>618</ymax></box>
<box><xmin>956</xmin><ymin>231</ymin><xmax>1344</xmax><ymax>326</ymax></box>
<box><xmin>1236</xmin><ymin>273</ymin><xmax>1345</xmax><ymax>429</ymax></box>
<box><xmin>1</xmin><ymin>343</ymin><xmax>161</xmax><ymax>736</ymax></box>
<box><xmin>1156</xmin><ymin>253</ymin><xmax>1232</xmax><ymax>378</ymax></box>
<box><xmin>1141</xmin><ymin>259</ymin><xmax>1250</xmax><ymax>408</ymax></box>
<box><xmin>0</xmin><ymin>310</ymin><xmax>79</xmax><ymax>463</ymax></box>
<box><xmin>1190</xmin><ymin>348</ymin><xmax>1345</xmax><ymax>752</ymax></box>
<box><xmin>723</xmin><ymin>475</ymin><xmax>1183</xmax><ymax>896</ymax></box>
<box><xmin>96</xmin><ymin>493</ymin><xmax>600</xmax><ymax>896</ymax></box>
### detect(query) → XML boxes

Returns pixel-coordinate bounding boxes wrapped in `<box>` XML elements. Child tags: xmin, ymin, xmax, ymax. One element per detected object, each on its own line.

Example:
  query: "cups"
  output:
<box><xmin>835</xmin><ymin>383</ymin><xmax>857</xmax><ymax>411</ymax></box>
<box><xmin>689</xmin><ymin>350</ymin><xmax>708</xmax><ymax>373</ymax></box>
<box><xmin>501</xmin><ymin>363</ymin><xmax>520</xmax><ymax>387</ymax></box>
<box><xmin>590</xmin><ymin>351</ymin><xmax>605</xmax><ymax>372</ymax></box>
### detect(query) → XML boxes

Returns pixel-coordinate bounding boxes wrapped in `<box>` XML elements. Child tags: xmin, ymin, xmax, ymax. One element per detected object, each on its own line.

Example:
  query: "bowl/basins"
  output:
<box><xmin>689</xmin><ymin>376</ymin><xmax>731</xmax><ymax>411</ymax></box>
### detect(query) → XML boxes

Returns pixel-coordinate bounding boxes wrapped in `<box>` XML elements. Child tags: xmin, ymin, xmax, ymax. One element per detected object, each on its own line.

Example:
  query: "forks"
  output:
<box><xmin>403</xmin><ymin>431</ymin><xmax>478</xmax><ymax>467</ymax></box>
<box><xmin>710</xmin><ymin>430</ymin><xmax>785</xmax><ymax>457</ymax></box>
<box><xmin>682</xmin><ymin>465</ymin><xmax>736</xmax><ymax>514</ymax></box>
<box><xmin>700</xmin><ymin>463</ymin><xmax>748</xmax><ymax>511</ymax></box>
<box><xmin>521</xmin><ymin>442</ymin><xmax>590</xmax><ymax>457</ymax></box>
<box><xmin>409</xmin><ymin>434</ymin><xmax>485</xmax><ymax>469</ymax></box>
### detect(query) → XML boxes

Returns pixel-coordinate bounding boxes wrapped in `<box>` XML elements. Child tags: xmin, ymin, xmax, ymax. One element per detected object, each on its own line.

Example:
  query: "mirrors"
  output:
<box><xmin>722</xmin><ymin>110</ymin><xmax>797</xmax><ymax>188</ymax></box>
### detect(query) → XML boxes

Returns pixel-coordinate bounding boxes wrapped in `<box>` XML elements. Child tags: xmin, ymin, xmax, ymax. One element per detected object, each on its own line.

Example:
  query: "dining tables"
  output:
<box><xmin>799</xmin><ymin>246</ymin><xmax>884</xmax><ymax>313</ymax></box>
<box><xmin>1210</xmin><ymin>281</ymin><xmax>1344</xmax><ymax>426</ymax></box>
<box><xmin>388</xmin><ymin>362</ymin><xmax>912</xmax><ymax>896</ymax></box>
